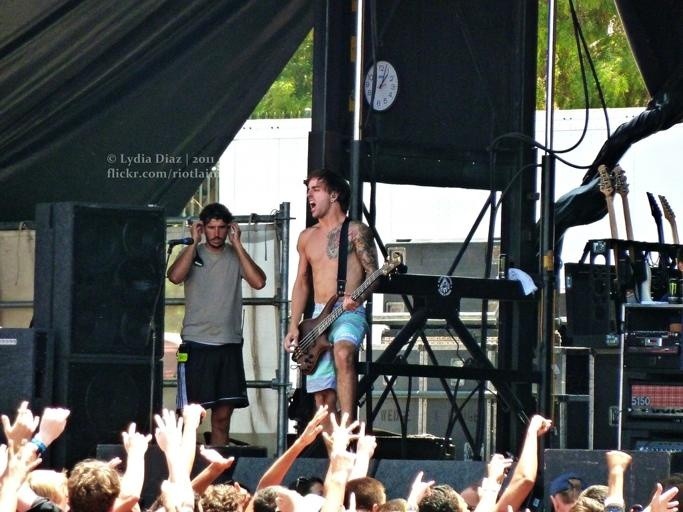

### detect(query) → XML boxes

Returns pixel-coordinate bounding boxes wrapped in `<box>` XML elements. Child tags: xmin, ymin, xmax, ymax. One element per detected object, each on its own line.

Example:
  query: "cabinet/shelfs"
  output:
<box><xmin>612</xmin><ymin>300</ymin><xmax>683</xmax><ymax>449</ymax></box>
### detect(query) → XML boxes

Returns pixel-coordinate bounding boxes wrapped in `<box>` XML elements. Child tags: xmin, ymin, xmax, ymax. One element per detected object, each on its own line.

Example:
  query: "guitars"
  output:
<box><xmin>292</xmin><ymin>254</ymin><xmax>404</xmax><ymax>375</ymax></box>
<box><xmin>600</xmin><ymin>162</ymin><xmax>683</xmax><ymax>302</ymax></box>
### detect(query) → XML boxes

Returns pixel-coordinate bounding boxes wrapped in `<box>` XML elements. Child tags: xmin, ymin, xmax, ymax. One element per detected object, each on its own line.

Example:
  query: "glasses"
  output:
<box><xmin>294</xmin><ymin>476</ymin><xmax>309</xmax><ymax>493</ymax></box>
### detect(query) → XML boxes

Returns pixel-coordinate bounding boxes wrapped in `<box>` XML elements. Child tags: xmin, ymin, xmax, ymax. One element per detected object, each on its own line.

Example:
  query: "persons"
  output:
<box><xmin>167</xmin><ymin>202</ymin><xmax>266</xmax><ymax>448</ymax></box>
<box><xmin>0</xmin><ymin>399</ymin><xmax>682</xmax><ymax>512</ymax></box>
<box><xmin>283</xmin><ymin>168</ymin><xmax>381</xmax><ymax>458</ymax></box>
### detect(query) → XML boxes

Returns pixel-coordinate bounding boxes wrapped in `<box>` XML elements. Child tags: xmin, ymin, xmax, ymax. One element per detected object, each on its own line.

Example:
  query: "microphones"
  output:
<box><xmin>166</xmin><ymin>237</ymin><xmax>195</xmax><ymax>246</ymax></box>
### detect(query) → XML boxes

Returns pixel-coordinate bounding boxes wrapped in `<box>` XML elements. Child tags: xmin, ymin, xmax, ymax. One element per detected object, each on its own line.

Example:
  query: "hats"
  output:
<box><xmin>547</xmin><ymin>471</ymin><xmax>589</xmax><ymax>498</ymax></box>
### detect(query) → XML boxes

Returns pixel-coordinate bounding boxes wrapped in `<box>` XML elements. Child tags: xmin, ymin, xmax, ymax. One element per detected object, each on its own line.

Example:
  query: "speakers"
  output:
<box><xmin>95</xmin><ymin>443</ymin><xmax>269</xmax><ymax>512</ymax></box>
<box><xmin>0</xmin><ymin>202</ymin><xmax>166</xmax><ymax>478</ymax></box>
<box><xmin>373</xmin><ymin>458</ymin><xmax>490</xmax><ymax>505</ymax></box>
<box><xmin>231</xmin><ymin>456</ymin><xmax>381</xmax><ymax>496</ymax></box>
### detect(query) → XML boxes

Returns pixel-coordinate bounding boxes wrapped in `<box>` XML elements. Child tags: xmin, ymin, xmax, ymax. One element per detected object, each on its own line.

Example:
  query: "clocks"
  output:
<box><xmin>361</xmin><ymin>59</ymin><xmax>401</xmax><ymax>114</ymax></box>
